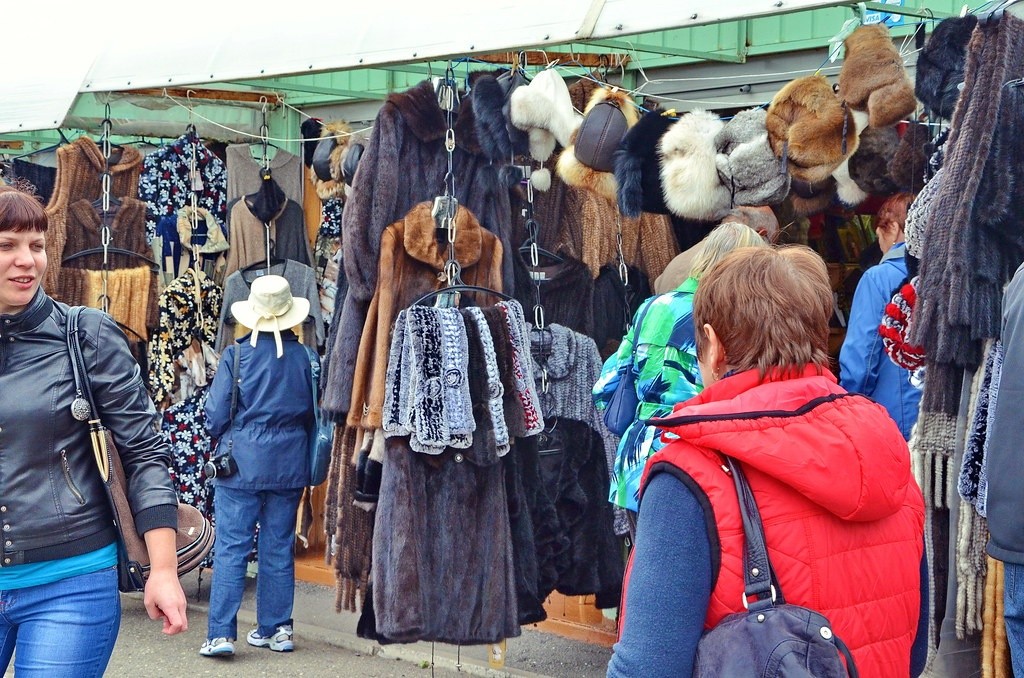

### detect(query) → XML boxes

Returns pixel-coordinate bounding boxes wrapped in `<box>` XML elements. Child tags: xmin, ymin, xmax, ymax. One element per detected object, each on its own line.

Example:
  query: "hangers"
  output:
<box><xmin>0</xmin><ymin>145</ymin><xmax>13</xmax><ymax>168</ymax></box>
<box><xmin>89</xmin><ymin>172</ymin><xmax>124</xmax><ymax>216</ymax></box>
<box><xmin>976</xmin><ymin>0</ymin><xmax>1007</xmax><ymax>31</ymax></box>
<box><xmin>95</xmin><ymin>292</ymin><xmax>151</xmax><ymax>361</ymax></box>
<box><xmin>518</xmin><ymin>217</ymin><xmax>566</xmax><ymax>265</ymax></box>
<box><xmin>13</xmin><ymin>125</ymin><xmax>73</xmax><ymax>163</ymax></box>
<box><xmin>249</xmin><ymin>124</ymin><xmax>279</xmax><ymax>161</ymax></box>
<box><xmin>244</xmin><ymin>166</ymin><xmax>272</xmax><ymax>200</ymax></box>
<box><xmin>61</xmin><ymin>223</ymin><xmax>161</xmax><ymax>274</ymax></box>
<box><xmin>186</xmin><ymin>122</ymin><xmax>198</xmax><ymax>144</ymax></box>
<box><xmin>91</xmin><ymin>118</ymin><xmax>126</xmax><ymax>167</ymax></box>
<box><xmin>537</xmin><ymin>389</ymin><xmax>565</xmax><ymax>456</ymax></box>
<box><xmin>426</xmin><ymin>52</ymin><xmax>612</xmax><ymax>95</ymax></box>
<box><xmin>432</xmin><ymin>172</ymin><xmax>459</xmax><ymax>220</ymax></box>
<box><xmin>992</xmin><ymin>0</ymin><xmax>1024</xmax><ymax>30</ymax></box>
<box><xmin>241</xmin><ymin>238</ymin><xmax>286</xmax><ymax>275</ymax></box>
<box><xmin>528</xmin><ymin>303</ymin><xmax>553</xmax><ymax>356</ymax></box>
<box><xmin>412</xmin><ymin>260</ymin><xmax>515</xmax><ymax>313</ymax></box>
<box><xmin>611</xmin><ymin>234</ymin><xmax>634</xmax><ymax>276</ymax></box>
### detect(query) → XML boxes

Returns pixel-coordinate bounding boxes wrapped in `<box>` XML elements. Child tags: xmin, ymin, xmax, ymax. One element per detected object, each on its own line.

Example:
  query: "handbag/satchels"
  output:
<box><xmin>309</xmin><ymin>419</ymin><xmax>333</xmax><ymax>484</ymax></box>
<box><xmin>65</xmin><ymin>306</ymin><xmax>217</xmax><ymax>593</ymax></box>
<box><xmin>693</xmin><ymin>454</ymin><xmax>858</xmax><ymax>678</ymax></box>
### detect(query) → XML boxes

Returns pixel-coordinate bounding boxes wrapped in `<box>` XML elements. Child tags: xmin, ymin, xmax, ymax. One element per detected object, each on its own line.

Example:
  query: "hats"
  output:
<box><xmin>229</xmin><ymin>274</ymin><xmax>311</xmax><ymax>359</ymax></box>
<box><xmin>473</xmin><ymin>13</ymin><xmax>979</xmax><ymax>221</ymax></box>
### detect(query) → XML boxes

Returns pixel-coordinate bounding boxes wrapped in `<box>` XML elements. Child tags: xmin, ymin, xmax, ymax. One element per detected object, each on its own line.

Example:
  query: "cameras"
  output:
<box><xmin>205</xmin><ymin>452</ymin><xmax>238</xmax><ymax>479</ymax></box>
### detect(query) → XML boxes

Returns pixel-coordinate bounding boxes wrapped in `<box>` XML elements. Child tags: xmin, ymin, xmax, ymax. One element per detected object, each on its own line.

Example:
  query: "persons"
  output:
<box><xmin>837</xmin><ymin>192</ymin><xmax>923</xmax><ymax>446</ymax></box>
<box><xmin>984</xmin><ymin>255</ymin><xmax>1024</xmax><ymax>678</ymax></box>
<box><xmin>605</xmin><ymin>244</ymin><xmax>930</xmax><ymax>678</ymax></box>
<box><xmin>652</xmin><ymin>204</ymin><xmax>780</xmax><ymax>294</ymax></box>
<box><xmin>591</xmin><ymin>219</ymin><xmax>771</xmax><ymax>548</ymax></box>
<box><xmin>0</xmin><ymin>180</ymin><xmax>189</xmax><ymax>678</ymax></box>
<box><xmin>198</xmin><ymin>271</ymin><xmax>324</xmax><ymax>660</ymax></box>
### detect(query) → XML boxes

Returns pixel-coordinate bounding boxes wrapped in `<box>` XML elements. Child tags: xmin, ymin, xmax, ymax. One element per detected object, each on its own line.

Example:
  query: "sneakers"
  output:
<box><xmin>198</xmin><ymin>637</ymin><xmax>237</xmax><ymax>657</ymax></box>
<box><xmin>245</xmin><ymin>623</ymin><xmax>293</xmax><ymax>651</ymax></box>
